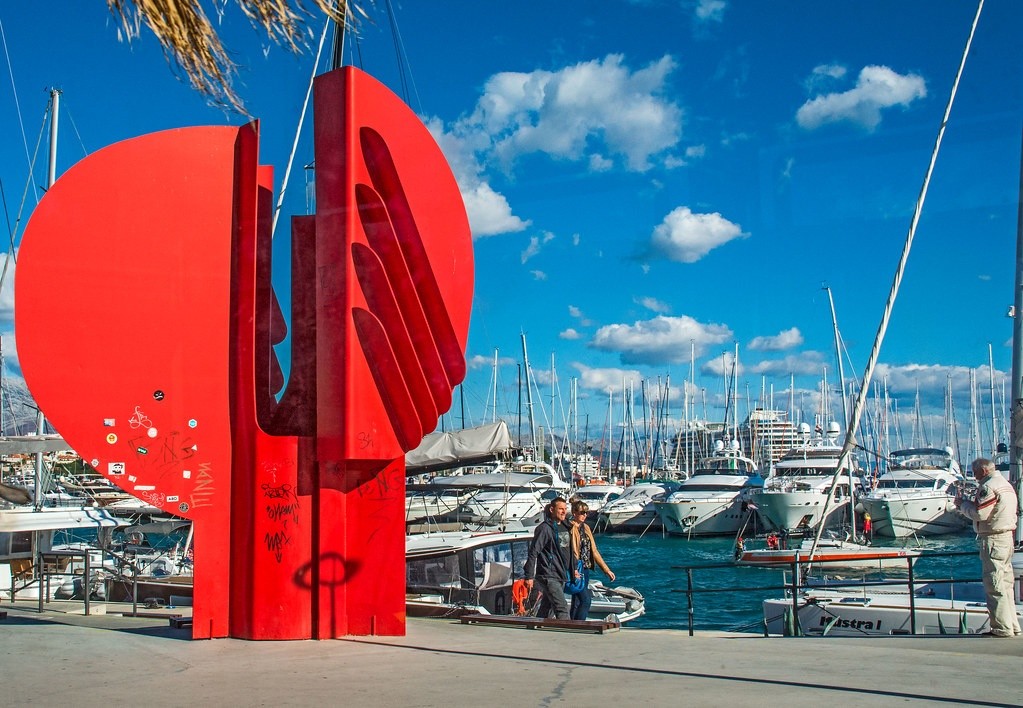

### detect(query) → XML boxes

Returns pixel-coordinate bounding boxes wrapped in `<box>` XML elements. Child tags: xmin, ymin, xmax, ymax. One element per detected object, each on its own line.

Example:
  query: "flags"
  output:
<box><xmin>741</xmin><ymin>495</ymin><xmax>758</xmax><ymax>512</ymax></box>
<box><xmin>573</xmin><ymin>473</ymin><xmax>582</xmax><ymax>482</ymax></box>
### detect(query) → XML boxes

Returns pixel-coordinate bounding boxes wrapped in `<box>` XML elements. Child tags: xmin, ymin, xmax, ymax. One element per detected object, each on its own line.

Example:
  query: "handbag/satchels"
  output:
<box><xmin>563</xmin><ymin>560</ymin><xmax>586</xmax><ymax>594</ymax></box>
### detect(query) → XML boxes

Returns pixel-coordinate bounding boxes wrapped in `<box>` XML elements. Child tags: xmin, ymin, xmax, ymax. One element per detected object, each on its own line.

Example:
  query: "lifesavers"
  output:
<box><xmin>512</xmin><ymin>580</ymin><xmax>528</xmax><ymax>605</ymax></box>
<box><xmin>130</xmin><ymin>530</ymin><xmax>143</xmax><ymax>544</ymax></box>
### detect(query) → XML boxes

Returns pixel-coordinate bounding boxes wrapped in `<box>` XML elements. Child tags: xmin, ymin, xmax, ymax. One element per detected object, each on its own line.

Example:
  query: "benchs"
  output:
<box><xmin>459</xmin><ymin>614</ymin><xmax>621</xmax><ymax>635</ymax></box>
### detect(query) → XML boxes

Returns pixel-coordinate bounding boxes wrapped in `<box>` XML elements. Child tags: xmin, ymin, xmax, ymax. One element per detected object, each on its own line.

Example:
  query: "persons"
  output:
<box><xmin>954</xmin><ymin>458</ymin><xmax>1022</xmax><ymax>638</ymax></box>
<box><xmin>178</xmin><ymin>546</ymin><xmax>194</xmax><ymax>575</ymax></box>
<box><xmin>738</xmin><ymin>527</ymin><xmax>789</xmax><ymax>551</ymax></box>
<box><xmin>863</xmin><ymin>512</ymin><xmax>872</xmax><ymax>546</ymax></box>
<box><xmin>567</xmin><ymin>497</ymin><xmax>616</xmax><ymax>621</ymax></box>
<box><xmin>141</xmin><ymin>559</ymin><xmax>147</xmax><ymax>574</ymax></box>
<box><xmin>523</xmin><ymin>497</ymin><xmax>581</xmax><ymax>620</ymax></box>
<box><xmin>802</xmin><ymin>514</ymin><xmax>812</xmax><ymax>539</ymax></box>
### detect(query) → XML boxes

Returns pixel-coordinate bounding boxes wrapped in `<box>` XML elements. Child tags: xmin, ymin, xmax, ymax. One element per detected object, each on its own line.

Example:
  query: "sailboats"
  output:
<box><xmin>1</xmin><ymin>1</ymin><xmax>1023</xmax><ymax>632</ymax></box>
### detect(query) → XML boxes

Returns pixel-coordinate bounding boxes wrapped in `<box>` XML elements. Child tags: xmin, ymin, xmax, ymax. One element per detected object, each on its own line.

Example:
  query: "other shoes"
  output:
<box><xmin>982</xmin><ymin>632</ymin><xmax>1009</xmax><ymax>637</ymax></box>
<box><xmin>1013</xmin><ymin>632</ymin><xmax>1022</xmax><ymax>636</ymax></box>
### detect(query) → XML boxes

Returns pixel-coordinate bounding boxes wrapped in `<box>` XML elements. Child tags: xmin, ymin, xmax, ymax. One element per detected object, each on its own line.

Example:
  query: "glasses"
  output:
<box><xmin>578</xmin><ymin>511</ymin><xmax>588</xmax><ymax>515</ymax></box>
